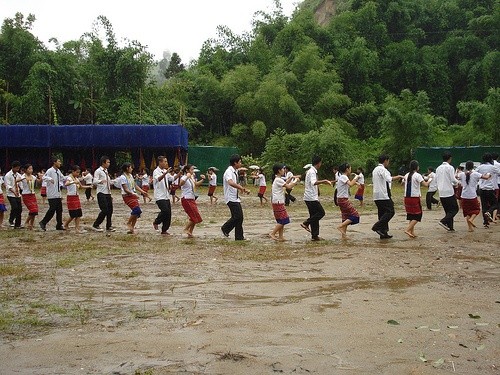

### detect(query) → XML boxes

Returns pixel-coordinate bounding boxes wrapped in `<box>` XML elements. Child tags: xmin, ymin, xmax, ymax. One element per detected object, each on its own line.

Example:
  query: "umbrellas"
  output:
<box><xmin>248</xmin><ymin>165</ymin><xmax>260</xmax><ymax>169</ymax></box>
<box><xmin>193</xmin><ymin>165</ymin><xmax>219</xmax><ymax>172</ymax></box>
<box><xmin>238</xmin><ymin>166</ymin><xmax>248</xmax><ymax>171</ymax></box>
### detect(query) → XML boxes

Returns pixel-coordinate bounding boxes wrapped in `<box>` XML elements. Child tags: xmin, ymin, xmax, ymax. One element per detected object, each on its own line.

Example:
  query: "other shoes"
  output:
<box><xmin>164</xmin><ymin>231</ymin><xmax>168</xmax><ymax>234</ymax></box>
<box><xmin>285</xmin><ymin>204</ymin><xmax>289</xmax><ymax>206</ymax></box>
<box><xmin>222</xmin><ymin>227</ymin><xmax>229</xmax><ymax>237</ymax></box>
<box><xmin>423</xmin><ymin>208</ymin><xmax>430</xmax><ymax>211</ymax></box>
<box><xmin>153</xmin><ymin>222</ymin><xmax>158</xmax><ymax>229</ymax></box>
<box><xmin>301</xmin><ymin>222</ymin><xmax>311</xmax><ymax>232</ymax></box>
<box><xmin>436</xmin><ymin>201</ymin><xmax>440</xmax><ymax>208</ymax></box>
<box><xmin>15</xmin><ymin>226</ymin><xmax>21</xmax><ymax>228</ymax></box>
<box><xmin>312</xmin><ymin>237</ymin><xmax>324</xmax><ymax>241</ymax></box>
<box><xmin>380</xmin><ymin>234</ymin><xmax>393</xmax><ymax>239</ymax></box>
<box><xmin>438</xmin><ymin>221</ymin><xmax>455</xmax><ymax>232</ymax></box>
<box><xmin>10</xmin><ymin>222</ymin><xmax>14</xmax><ymax>227</ymax></box>
<box><xmin>57</xmin><ymin>226</ymin><xmax>66</xmax><ymax>230</ymax></box>
<box><xmin>195</xmin><ymin>196</ymin><xmax>198</xmax><ymax>200</ymax></box>
<box><xmin>235</xmin><ymin>239</ymin><xmax>246</xmax><ymax>242</ymax></box>
<box><xmin>92</xmin><ymin>196</ymin><xmax>94</xmax><ymax>200</ymax></box>
<box><xmin>372</xmin><ymin>226</ymin><xmax>386</xmax><ymax>236</ymax></box>
<box><xmin>481</xmin><ymin>211</ymin><xmax>500</xmax><ymax>228</ymax></box>
<box><xmin>107</xmin><ymin>227</ymin><xmax>116</xmax><ymax>232</ymax></box>
<box><xmin>93</xmin><ymin>226</ymin><xmax>104</xmax><ymax>232</ymax></box>
<box><xmin>39</xmin><ymin>221</ymin><xmax>46</xmax><ymax>231</ymax></box>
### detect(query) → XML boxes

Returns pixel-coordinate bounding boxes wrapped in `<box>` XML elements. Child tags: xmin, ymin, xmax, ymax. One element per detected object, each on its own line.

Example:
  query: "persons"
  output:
<box><xmin>168</xmin><ymin>168</ymin><xmax>218</xmax><ymax>205</ymax></box>
<box><xmin>238</xmin><ymin>168</ymin><xmax>260</xmax><ymax>186</ymax></box>
<box><xmin>250</xmin><ymin>169</ymin><xmax>268</xmax><ymax>206</ymax></box>
<box><xmin>435</xmin><ymin>152</ymin><xmax>500</xmax><ymax>232</ymax></box>
<box><xmin>423</xmin><ymin>168</ymin><xmax>440</xmax><ymax>210</ymax></box>
<box><xmin>92</xmin><ymin>156</ymin><xmax>205</xmax><ymax>238</ymax></box>
<box><xmin>281</xmin><ymin>164</ymin><xmax>319</xmax><ymax>206</ymax></box>
<box><xmin>300</xmin><ymin>157</ymin><xmax>332</xmax><ymax>241</ymax></box>
<box><xmin>335</xmin><ymin>163</ymin><xmax>362</xmax><ymax>240</ymax></box>
<box><xmin>222</xmin><ymin>154</ymin><xmax>251</xmax><ymax>242</ymax></box>
<box><xmin>352</xmin><ymin>167</ymin><xmax>365</xmax><ymax>207</ymax></box>
<box><xmin>326</xmin><ymin>167</ymin><xmax>341</xmax><ymax>207</ymax></box>
<box><xmin>0</xmin><ymin>156</ymin><xmax>95</xmax><ymax>234</ymax></box>
<box><xmin>371</xmin><ymin>154</ymin><xmax>406</xmax><ymax>239</ymax></box>
<box><xmin>269</xmin><ymin>164</ymin><xmax>299</xmax><ymax>241</ymax></box>
<box><xmin>402</xmin><ymin>160</ymin><xmax>432</xmax><ymax>237</ymax></box>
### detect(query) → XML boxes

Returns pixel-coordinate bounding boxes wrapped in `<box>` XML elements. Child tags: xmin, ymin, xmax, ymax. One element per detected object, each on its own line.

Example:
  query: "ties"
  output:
<box><xmin>234</xmin><ymin>172</ymin><xmax>238</xmax><ymax>199</ymax></box>
<box><xmin>13</xmin><ymin>174</ymin><xmax>19</xmax><ymax>196</ymax></box>
<box><xmin>56</xmin><ymin>171</ymin><xmax>59</xmax><ymax>192</ymax></box>
<box><xmin>103</xmin><ymin>170</ymin><xmax>109</xmax><ymax>191</ymax></box>
<box><xmin>161</xmin><ymin>171</ymin><xmax>167</xmax><ymax>189</ymax></box>
<box><xmin>316</xmin><ymin>173</ymin><xmax>320</xmax><ymax>196</ymax></box>
